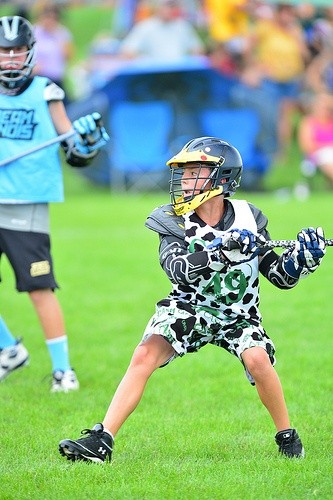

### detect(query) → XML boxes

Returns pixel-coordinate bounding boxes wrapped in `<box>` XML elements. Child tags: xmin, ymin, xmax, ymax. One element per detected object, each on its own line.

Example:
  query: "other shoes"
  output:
<box><xmin>50</xmin><ymin>369</ymin><xmax>79</xmax><ymax>393</ymax></box>
<box><xmin>0</xmin><ymin>337</ymin><xmax>30</xmax><ymax>381</ymax></box>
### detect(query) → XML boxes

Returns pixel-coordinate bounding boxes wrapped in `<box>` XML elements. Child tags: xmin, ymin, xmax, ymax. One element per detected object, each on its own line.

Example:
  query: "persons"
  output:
<box><xmin>58</xmin><ymin>137</ymin><xmax>324</xmax><ymax>465</ymax></box>
<box><xmin>1</xmin><ymin>16</ymin><xmax>107</xmax><ymax>396</ymax></box>
<box><xmin>14</xmin><ymin>0</ymin><xmax>332</xmax><ymax>181</ymax></box>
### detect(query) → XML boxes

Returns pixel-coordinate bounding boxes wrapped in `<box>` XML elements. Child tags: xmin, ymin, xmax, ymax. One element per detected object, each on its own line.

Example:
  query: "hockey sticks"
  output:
<box><xmin>1</xmin><ymin>124</ymin><xmax>84</xmax><ymax>169</ymax></box>
<box><xmin>228</xmin><ymin>240</ymin><xmax>333</xmax><ymax>251</ymax></box>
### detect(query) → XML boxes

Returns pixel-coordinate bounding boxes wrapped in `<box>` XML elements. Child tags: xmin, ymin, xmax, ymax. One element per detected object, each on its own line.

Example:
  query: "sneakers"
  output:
<box><xmin>275</xmin><ymin>428</ymin><xmax>306</xmax><ymax>460</ymax></box>
<box><xmin>59</xmin><ymin>422</ymin><xmax>114</xmax><ymax>465</ymax></box>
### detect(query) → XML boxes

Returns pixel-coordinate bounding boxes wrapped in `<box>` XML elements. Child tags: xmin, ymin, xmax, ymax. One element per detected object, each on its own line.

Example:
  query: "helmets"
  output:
<box><xmin>167</xmin><ymin>137</ymin><xmax>242</xmax><ymax>216</ymax></box>
<box><xmin>0</xmin><ymin>16</ymin><xmax>37</xmax><ymax>96</ymax></box>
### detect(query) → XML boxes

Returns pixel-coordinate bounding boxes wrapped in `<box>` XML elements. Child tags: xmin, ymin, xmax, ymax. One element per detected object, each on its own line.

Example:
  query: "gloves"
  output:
<box><xmin>72</xmin><ymin>112</ymin><xmax>110</xmax><ymax>154</ymax></box>
<box><xmin>206</xmin><ymin>228</ymin><xmax>267</xmax><ymax>273</ymax></box>
<box><xmin>282</xmin><ymin>227</ymin><xmax>326</xmax><ymax>279</ymax></box>
